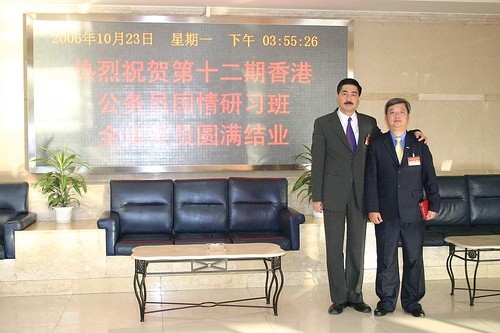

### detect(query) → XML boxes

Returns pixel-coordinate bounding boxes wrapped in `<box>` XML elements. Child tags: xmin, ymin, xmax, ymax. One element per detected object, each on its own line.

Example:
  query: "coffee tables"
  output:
<box><xmin>132</xmin><ymin>242</ymin><xmax>286</xmax><ymax>322</ymax></box>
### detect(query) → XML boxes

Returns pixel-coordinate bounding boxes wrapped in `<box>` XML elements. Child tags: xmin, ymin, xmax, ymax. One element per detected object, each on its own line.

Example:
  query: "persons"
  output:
<box><xmin>312</xmin><ymin>78</ymin><xmax>427</xmax><ymax>314</ymax></box>
<box><xmin>366</xmin><ymin>98</ymin><xmax>442</xmax><ymax>317</ymax></box>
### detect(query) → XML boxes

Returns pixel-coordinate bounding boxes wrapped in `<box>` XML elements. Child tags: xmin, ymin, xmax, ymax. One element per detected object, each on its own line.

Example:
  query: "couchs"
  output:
<box><xmin>97</xmin><ymin>177</ymin><xmax>306</xmax><ymax>255</ymax></box>
<box><xmin>0</xmin><ymin>182</ymin><xmax>37</xmax><ymax>259</ymax></box>
<box><xmin>399</xmin><ymin>174</ymin><xmax>500</xmax><ymax>248</ymax></box>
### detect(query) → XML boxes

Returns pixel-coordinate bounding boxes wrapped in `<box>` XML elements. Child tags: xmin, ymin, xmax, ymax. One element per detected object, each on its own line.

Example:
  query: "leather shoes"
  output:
<box><xmin>412</xmin><ymin>308</ymin><xmax>425</xmax><ymax>317</ymax></box>
<box><xmin>374</xmin><ymin>306</ymin><xmax>387</xmax><ymax>316</ymax></box>
<box><xmin>347</xmin><ymin>301</ymin><xmax>372</xmax><ymax>313</ymax></box>
<box><xmin>328</xmin><ymin>303</ymin><xmax>347</xmax><ymax>315</ymax></box>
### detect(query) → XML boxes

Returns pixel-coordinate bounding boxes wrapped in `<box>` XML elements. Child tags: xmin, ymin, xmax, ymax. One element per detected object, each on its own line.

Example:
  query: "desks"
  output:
<box><xmin>444</xmin><ymin>234</ymin><xmax>500</xmax><ymax>305</ymax></box>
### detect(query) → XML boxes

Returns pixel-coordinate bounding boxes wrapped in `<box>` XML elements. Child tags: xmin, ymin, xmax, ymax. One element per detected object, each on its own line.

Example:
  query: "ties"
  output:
<box><xmin>345</xmin><ymin>118</ymin><xmax>357</xmax><ymax>154</ymax></box>
<box><xmin>394</xmin><ymin>138</ymin><xmax>404</xmax><ymax>165</ymax></box>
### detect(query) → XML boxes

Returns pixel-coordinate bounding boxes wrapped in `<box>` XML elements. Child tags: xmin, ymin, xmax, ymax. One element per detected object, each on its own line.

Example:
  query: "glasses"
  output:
<box><xmin>385</xmin><ymin>110</ymin><xmax>407</xmax><ymax>118</ymax></box>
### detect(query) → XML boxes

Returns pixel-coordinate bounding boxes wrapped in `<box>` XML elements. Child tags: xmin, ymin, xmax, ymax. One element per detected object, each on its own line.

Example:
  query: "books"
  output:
<box><xmin>420</xmin><ymin>200</ymin><xmax>429</xmax><ymax>219</ymax></box>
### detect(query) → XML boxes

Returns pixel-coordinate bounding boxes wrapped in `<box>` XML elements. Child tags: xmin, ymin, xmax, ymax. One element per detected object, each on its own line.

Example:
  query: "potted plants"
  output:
<box><xmin>31</xmin><ymin>145</ymin><xmax>95</xmax><ymax>224</ymax></box>
<box><xmin>293</xmin><ymin>144</ymin><xmax>324</xmax><ymax>218</ymax></box>
